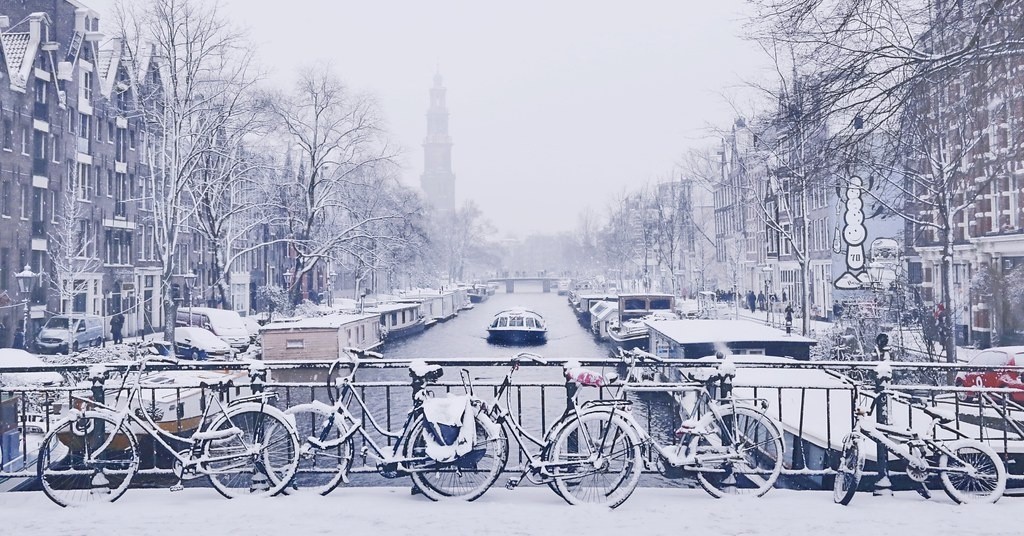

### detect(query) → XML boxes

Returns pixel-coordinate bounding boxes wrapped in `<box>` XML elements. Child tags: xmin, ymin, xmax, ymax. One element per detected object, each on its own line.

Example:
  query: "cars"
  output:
<box><xmin>173</xmin><ymin>326</ymin><xmax>233</xmax><ymax>360</ymax></box>
<box><xmin>953</xmin><ymin>346</ymin><xmax>1024</xmax><ymax>403</ymax></box>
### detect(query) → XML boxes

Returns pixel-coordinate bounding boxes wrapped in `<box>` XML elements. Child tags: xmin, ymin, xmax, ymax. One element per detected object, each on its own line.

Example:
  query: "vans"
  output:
<box><xmin>36</xmin><ymin>313</ymin><xmax>104</xmax><ymax>355</ymax></box>
<box><xmin>175</xmin><ymin>307</ymin><xmax>251</xmax><ymax>353</ymax></box>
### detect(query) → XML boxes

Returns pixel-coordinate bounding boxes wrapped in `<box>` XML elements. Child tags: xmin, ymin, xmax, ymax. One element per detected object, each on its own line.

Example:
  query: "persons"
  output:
<box><xmin>749</xmin><ymin>291</ymin><xmax>756</xmax><ymax>313</ymax></box>
<box><xmin>12</xmin><ymin>326</ymin><xmax>24</xmax><ymax>349</ymax></box>
<box><xmin>784</xmin><ymin>304</ymin><xmax>794</xmax><ymax>323</ymax></box>
<box><xmin>757</xmin><ymin>290</ymin><xmax>766</xmax><ymax>311</ymax></box>
<box><xmin>747</xmin><ymin>290</ymin><xmax>752</xmax><ymax>310</ymax></box>
<box><xmin>491</xmin><ymin>267</ymin><xmax>580</xmax><ymax>278</ymax></box>
<box><xmin>109</xmin><ymin>307</ymin><xmax>125</xmax><ymax>344</ymax></box>
<box><xmin>833</xmin><ymin>300</ymin><xmax>844</xmax><ymax>328</ymax></box>
<box><xmin>715</xmin><ymin>290</ymin><xmax>743</xmax><ymax>302</ymax></box>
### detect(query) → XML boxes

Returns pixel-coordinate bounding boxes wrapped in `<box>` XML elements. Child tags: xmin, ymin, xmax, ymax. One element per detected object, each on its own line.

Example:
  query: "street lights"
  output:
<box><xmin>16</xmin><ymin>264</ymin><xmax>38</xmax><ymax>352</ymax></box>
<box><xmin>761</xmin><ymin>264</ymin><xmax>774</xmax><ymax>322</ymax></box>
<box><xmin>184</xmin><ymin>267</ymin><xmax>198</xmax><ymax>326</ymax></box>
<box><xmin>330</xmin><ymin>271</ymin><xmax>339</xmax><ymax>308</ymax></box>
<box><xmin>693</xmin><ymin>266</ymin><xmax>702</xmax><ymax>319</ymax></box>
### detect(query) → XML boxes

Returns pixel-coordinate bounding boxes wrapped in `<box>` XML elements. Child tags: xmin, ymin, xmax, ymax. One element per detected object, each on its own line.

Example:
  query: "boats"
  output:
<box><xmin>565</xmin><ymin>288</ymin><xmax>1024</xmax><ymax>489</ymax></box>
<box><xmin>486</xmin><ymin>307</ymin><xmax>548</xmax><ymax>343</ymax></box>
<box><xmin>259</xmin><ymin>283</ymin><xmax>496</xmax><ymax>367</ymax></box>
<box><xmin>52</xmin><ymin>358</ymin><xmax>280</xmax><ymax>459</ymax></box>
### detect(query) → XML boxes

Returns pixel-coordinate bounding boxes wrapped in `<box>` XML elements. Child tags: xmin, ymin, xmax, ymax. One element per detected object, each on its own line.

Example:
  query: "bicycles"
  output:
<box><xmin>38</xmin><ymin>356</ymin><xmax>997</xmax><ymax>507</ymax></box>
